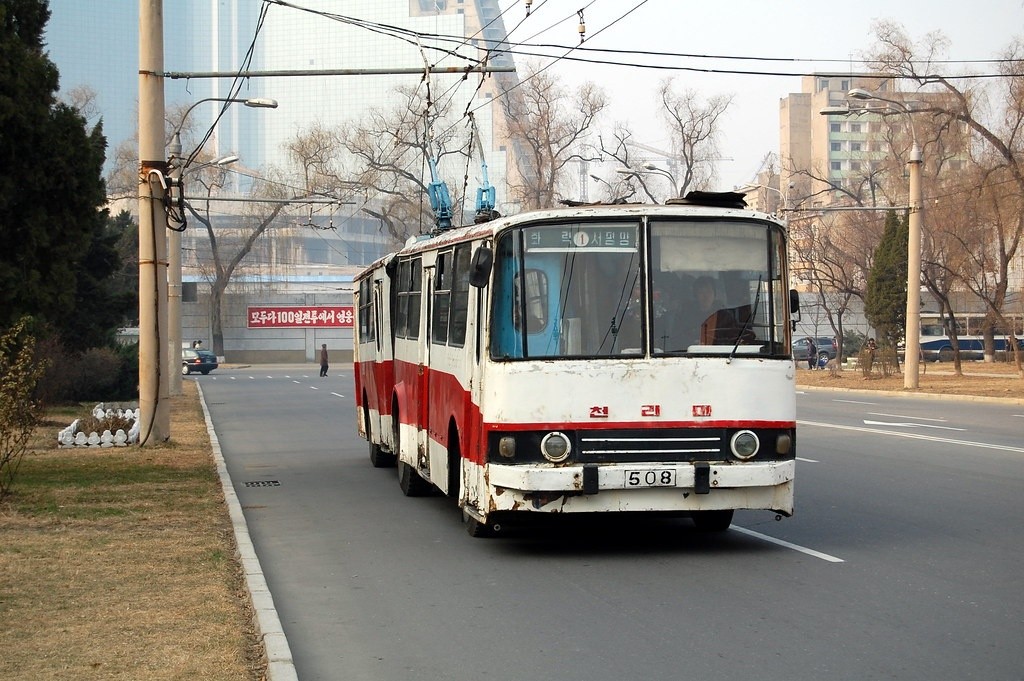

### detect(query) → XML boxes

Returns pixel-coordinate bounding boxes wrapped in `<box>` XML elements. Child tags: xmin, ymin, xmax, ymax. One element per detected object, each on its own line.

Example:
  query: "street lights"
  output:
<box><xmin>818</xmin><ymin>85</ymin><xmax>924</xmax><ymax>394</ymax></box>
<box><xmin>170</xmin><ymin>98</ymin><xmax>278</xmax><ymax>393</ymax></box>
<box><xmin>744</xmin><ymin>179</ymin><xmax>794</xmax><ymax>360</ymax></box>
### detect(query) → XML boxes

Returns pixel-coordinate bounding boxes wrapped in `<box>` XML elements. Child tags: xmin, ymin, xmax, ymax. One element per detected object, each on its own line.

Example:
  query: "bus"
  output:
<box><xmin>350</xmin><ymin>98</ymin><xmax>803</xmax><ymax>538</ymax></box>
<box><xmin>894</xmin><ymin>312</ymin><xmax>1024</xmax><ymax>363</ymax></box>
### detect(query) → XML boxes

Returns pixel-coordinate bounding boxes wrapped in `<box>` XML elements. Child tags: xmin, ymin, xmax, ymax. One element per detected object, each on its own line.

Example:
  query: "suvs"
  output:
<box><xmin>792</xmin><ymin>336</ymin><xmax>837</xmax><ymax>365</ymax></box>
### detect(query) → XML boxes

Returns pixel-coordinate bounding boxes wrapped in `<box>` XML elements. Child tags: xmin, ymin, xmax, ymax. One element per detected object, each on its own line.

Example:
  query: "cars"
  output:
<box><xmin>182</xmin><ymin>346</ymin><xmax>221</xmax><ymax>377</ymax></box>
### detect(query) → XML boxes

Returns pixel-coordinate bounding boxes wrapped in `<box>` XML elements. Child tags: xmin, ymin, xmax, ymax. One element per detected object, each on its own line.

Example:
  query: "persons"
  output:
<box><xmin>193</xmin><ymin>340</ymin><xmax>202</xmax><ymax>349</ymax></box>
<box><xmin>806</xmin><ymin>338</ymin><xmax>816</xmax><ymax>370</ymax></box>
<box><xmin>319</xmin><ymin>344</ymin><xmax>329</xmax><ymax>377</ymax></box>
<box><xmin>867</xmin><ymin>338</ymin><xmax>876</xmax><ymax>370</ymax></box>
<box><xmin>670</xmin><ymin>276</ymin><xmax>756</xmax><ymax>353</ymax></box>
